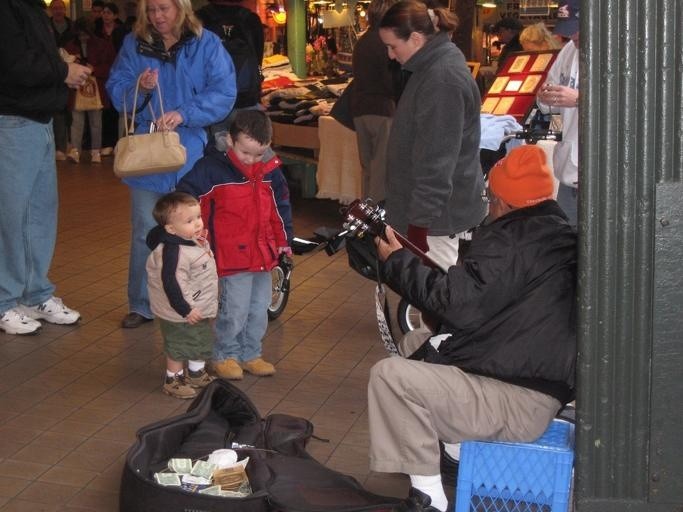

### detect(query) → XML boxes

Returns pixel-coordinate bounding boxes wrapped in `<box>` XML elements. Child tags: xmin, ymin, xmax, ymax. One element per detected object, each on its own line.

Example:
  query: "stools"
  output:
<box><xmin>454</xmin><ymin>420</ymin><xmax>575</xmax><ymax>512</ymax></box>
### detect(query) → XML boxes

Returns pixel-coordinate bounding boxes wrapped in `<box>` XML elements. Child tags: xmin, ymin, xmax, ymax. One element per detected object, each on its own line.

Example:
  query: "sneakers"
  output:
<box><xmin>0</xmin><ymin>307</ymin><xmax>42</xmax><ymax>335</ymax></box>
<box><xmin>162</xmin><ymin>374</ymin><xmax>196</xmax><ymax>400</ymax></box>
<box><xmin>213</xmin><ymin>359</ymin><xmax>243</xmax><ymax>381</ymax></box>
<box><xmin>185</xmin><ymin>369</ymin><xmax>217</xmax><ymax>388</ymax></box>
<box><xmin>66</xmin><ymin>148</ymin><xmax>80</xmax><ymax>163</ymax></box>
<box><xmin>239</xmin><ymin>358</ymin><xmax>276</xmax><ymax>376</ymax></box>
<box><xmin>19</xmin><ymin>296</ymin><xmax>81</xmax><ymax>325</ymax></box>
<box><xmin>437</xmin><ymin>451</ymin><xmax>460</xmax><ymax>477</ymax></box>
<box><xmin>405</xmin><ymin>486</ymin><xmax>452</xmax><ymax>512</ymax></box>
<box><xmin>90</xmin><ymin>149</ymin><xmax>101</xmax><ymax>163</ymax></box>
<box><xmin>101</xmin><ymin>147</ymin><xmax>112</xmax><ymax>156</ymax></box>
<box><xmin>54</xmin><ymin>151</ymin><xmax>65</xmax><ymax>161</ymax></box>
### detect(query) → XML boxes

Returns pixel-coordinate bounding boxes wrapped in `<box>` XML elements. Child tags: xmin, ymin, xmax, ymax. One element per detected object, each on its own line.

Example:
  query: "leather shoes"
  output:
<box><xmin>120</xmin><ymin>312</ymin><xmax>153</xmax><ymax>328</ymax></box>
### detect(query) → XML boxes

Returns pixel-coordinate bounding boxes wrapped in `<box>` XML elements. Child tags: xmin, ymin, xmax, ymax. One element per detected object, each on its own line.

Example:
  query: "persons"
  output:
<box><xmin>105</xmin><ymin>0</ymin><xmax>237</xmax><ymax>328</ymax></box>
<box><xmin>48</xmin><ymin>1</ymin><xmax>76</xmax><ymax>161</ymax></box>
<box><xmin>495</xmin><ymin>18</ymin><xmax>523</xmax><ymax>71</ymax></box>
<box><xmin>196</xmin><ymin>0</ymin><xmax>265</xmax><ymax>132</ymax></box>
<box><xmin>0</xmin><ymin>0</ymin><xmax>91</xmax><ymax>336</ymax></box>
<box><xmin>96</xmin><ymin>3</ymin><xmax>126</xmax><ymax>157</ymax></box>
<box><xmin>351</xmin><ymin>1</ymin><xmax>413</xmax><ymax>206</ymax></box>
<box><xmin>519</xmin><ymin>22</ymin><xmax>561</xmax><ymax>53</ymax></box>
<box><xmin>76</xmin><ymin>0</ymin><xmax>104</xmax><ymax>38</ymax></box>
<box><xmin>143</xmin><ymin>192</ymin><xmax>219</xmax><ymax>400</ymax></box>
<box><xmin>535</xmin><ymin>1</ymin><xmax>581</xmax><ymax>231</ymax></box>
<box><xmin>175</xmin><ymin>109</ymin><xmax>294</xmax><ymax>381</ymax></box>
<box><xmin>366</xmin><ymin>145</ymin><xmax>579</xmax><ymax>512</ymax></box>
<box><xmin>63</xmin><ymin>17</ymin><xmax>117</xmax><ymax>163</ymax></box>
<box><xmin>378</xmin><ymin>0</ymin><xmax>489</xmax><ymax>334</ymax></box>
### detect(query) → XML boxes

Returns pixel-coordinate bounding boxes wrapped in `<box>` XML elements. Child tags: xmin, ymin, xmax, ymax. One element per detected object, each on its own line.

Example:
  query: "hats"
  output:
<box><xmin>551</xmin><ymin>0</ymin><xmax>580</xmax><ymax>38</ymax></box>
<box><xmin>486</xmin><ymin>143</ymin><xmax>555</xmax><ymax>208</ymax></box>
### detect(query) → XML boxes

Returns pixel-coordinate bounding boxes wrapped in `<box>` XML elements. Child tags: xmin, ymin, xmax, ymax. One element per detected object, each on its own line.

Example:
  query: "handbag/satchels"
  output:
<box><xmin>112</xmin><ymin>75</ymin><xmax>187</xmax><ymax>178</ymax></box>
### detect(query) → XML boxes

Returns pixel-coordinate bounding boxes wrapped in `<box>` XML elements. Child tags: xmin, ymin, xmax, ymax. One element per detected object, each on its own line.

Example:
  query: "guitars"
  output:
<box><xmin>338</xmin><ymin>198</ymin><xmax>447</xmax><ymax>334</ymax></box>
